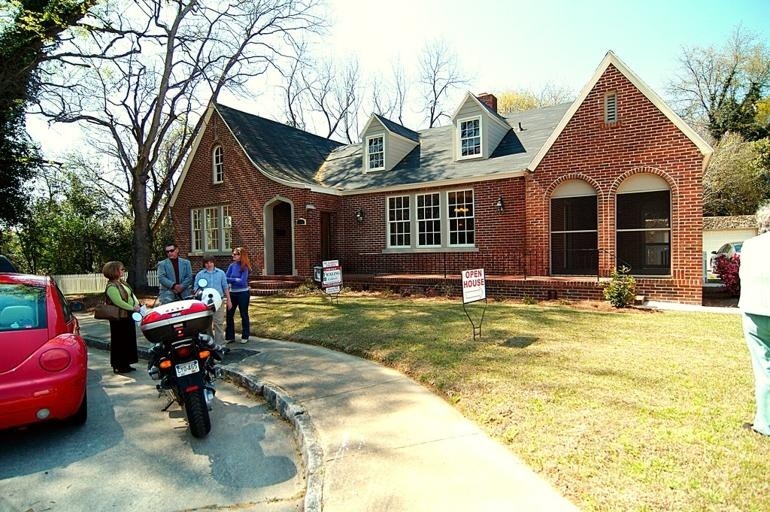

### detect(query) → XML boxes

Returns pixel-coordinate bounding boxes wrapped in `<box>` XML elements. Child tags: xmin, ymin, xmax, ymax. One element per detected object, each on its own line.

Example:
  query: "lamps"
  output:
<box><xmin>354</xmin><ymin>206</ymin><xmax>366</xmax><ymax>224</ymax></box>
<box><xmin>495</xmin><ymin>193</ymin><xmax>505</xmax><ymax>217</ymax></box>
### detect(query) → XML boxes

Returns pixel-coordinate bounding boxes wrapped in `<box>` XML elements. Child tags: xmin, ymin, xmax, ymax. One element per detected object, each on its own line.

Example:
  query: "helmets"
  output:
<box><xmin>202</xmin><ymin>288</ymin><xmax>222</xmax><ymax>312</ymax></box>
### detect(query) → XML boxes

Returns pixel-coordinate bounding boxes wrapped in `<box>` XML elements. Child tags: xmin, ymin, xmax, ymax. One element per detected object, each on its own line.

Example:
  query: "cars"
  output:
<box><xmin>0</xmin><ymin>271</ymin><xmax>91</xmax><ymax>438</ymax></box>
<box><xmin>709</xmin><ymin>241</ymin><xmax>743</xmax><ymax>272</ymax></box>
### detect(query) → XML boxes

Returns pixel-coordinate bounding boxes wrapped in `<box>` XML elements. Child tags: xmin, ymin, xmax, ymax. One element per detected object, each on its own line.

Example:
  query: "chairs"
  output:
<box><xmin>0</xmin><ymin>301</ymin><xmax>37</xmax><ymax>333</ymax></box>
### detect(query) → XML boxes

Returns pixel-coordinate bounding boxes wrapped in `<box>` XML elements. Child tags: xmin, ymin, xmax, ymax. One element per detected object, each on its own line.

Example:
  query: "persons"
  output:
<box><xmin>193</xmin><ymin>255</ymin><xmax>233</xmax><ymax>353</ymax></box>
<box><xmin>102</xmin><ymin>261</ymin><xmax>141</xmax><ymax>373</ymax></box>
<box><xmin>224</xmin><ymin>247</ymin><xmax>252</xmax><ymax>344</ymax></box>
<box><xmin>157</xmin><ymin>243</ymin><xmax>193</xmax><ymax>304</ymax></box>
<box><xmin>737</xmin><ymin>206</ymin><xmax>770</xmax><ymax>436</ymax></box>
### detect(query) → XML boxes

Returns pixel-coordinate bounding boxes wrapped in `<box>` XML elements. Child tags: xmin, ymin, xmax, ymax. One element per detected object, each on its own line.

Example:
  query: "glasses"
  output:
<box><xmin>166</xmin><ymin>248</ymin><xmax>176</xmax><ymax>253</ymax></box>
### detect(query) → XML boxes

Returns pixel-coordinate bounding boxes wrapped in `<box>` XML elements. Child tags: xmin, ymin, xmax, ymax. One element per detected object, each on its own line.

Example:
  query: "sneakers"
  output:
<box><xmin>113</xmin><ymin>365</ymin><xmax>136</xmax><ymax>373</ymax></box>
<box><xmin>225</xmin><ymin>339</ymin><xmax>235</xmax><ymax>343</ymax></box>
<box><xmin>241</xmin><ymin>339</ymin><xmax>248</xmax><ymax>343</ymax></box>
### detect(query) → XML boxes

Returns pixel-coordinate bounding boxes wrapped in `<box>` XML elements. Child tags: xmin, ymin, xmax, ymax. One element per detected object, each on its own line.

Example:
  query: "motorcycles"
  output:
<box><xmin>131</xmin><ymin>276</ymin><xmax>230</xmax><ymax>439</ymax></box>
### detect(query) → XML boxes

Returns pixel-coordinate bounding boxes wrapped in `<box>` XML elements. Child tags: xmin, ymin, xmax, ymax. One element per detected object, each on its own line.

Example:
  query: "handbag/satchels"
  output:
<box><xmin>95</xmin><ymin>304</ymin><xmax>120</xmax><ymax>320</ymax></box>
